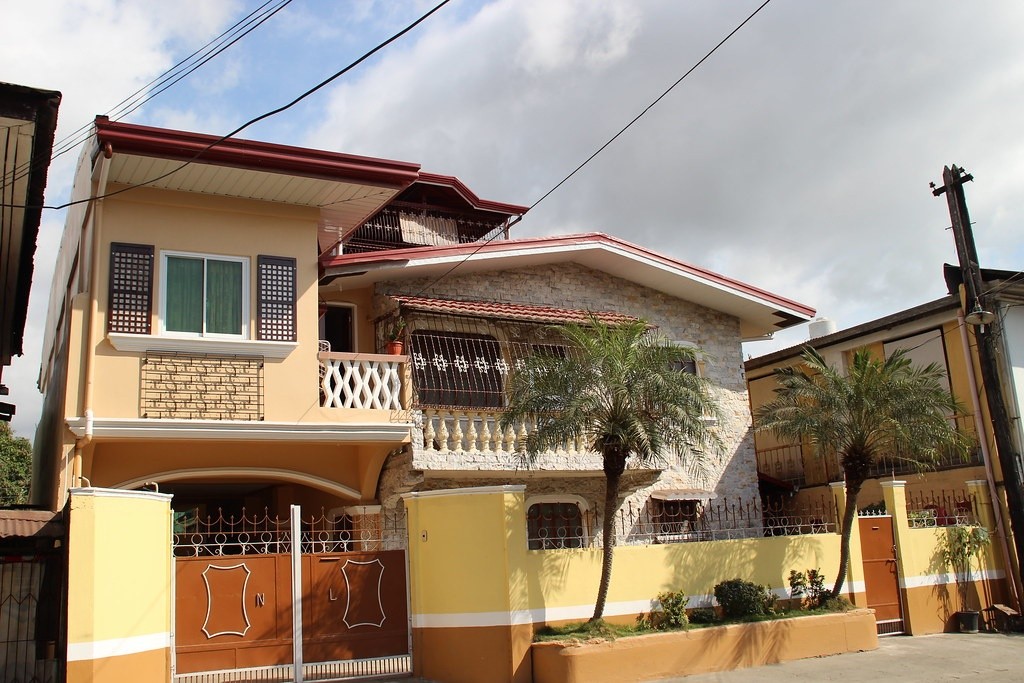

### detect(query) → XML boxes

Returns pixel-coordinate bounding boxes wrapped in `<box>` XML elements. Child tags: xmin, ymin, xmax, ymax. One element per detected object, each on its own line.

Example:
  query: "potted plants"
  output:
<box><xmin>382</xmin><ymin>317</ymin><xmax>406</xmax><ymax>355</ymax></box>
<box><xmin>934</xmin><ymin>525</ymin><xmax>992</xmax><ymax>634</ymax></box>
